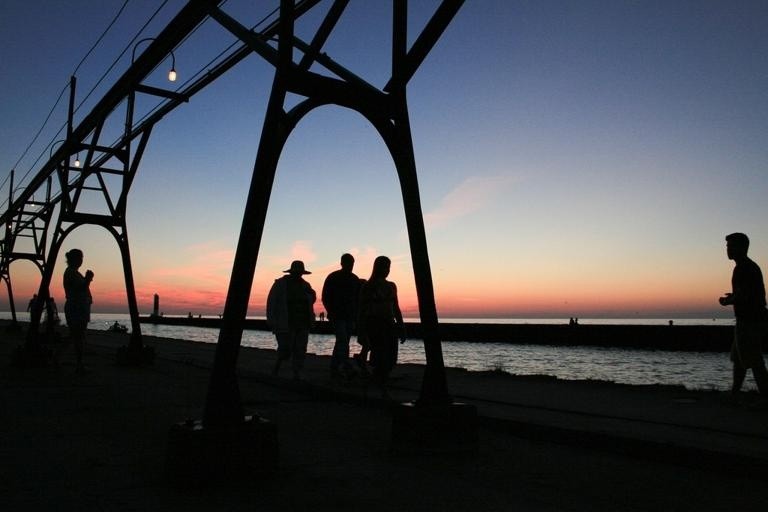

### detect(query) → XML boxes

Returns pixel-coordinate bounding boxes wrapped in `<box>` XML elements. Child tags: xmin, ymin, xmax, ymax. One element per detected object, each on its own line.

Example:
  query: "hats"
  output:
<box><xmin>283</xmin><ymin>261</ymin><xmax>311</xmax><ymax>274</ymax></box>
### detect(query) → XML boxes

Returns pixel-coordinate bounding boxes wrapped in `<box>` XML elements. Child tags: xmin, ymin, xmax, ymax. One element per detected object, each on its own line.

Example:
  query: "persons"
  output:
<box><xmin>44</xmin><ymin>298</ymin><xmax>58</xmax><ymax>316</ymax></box>
<box><xmin>353</xmin><ymin>279</ymin><xmax>368</xmax><ymax>361</ymax></box>
<box><xmin>356</xmin><ymin>256</ymin><xmax>407</xmax><ymax>371</ymax></box>
<box><xmin>321</xmin><ymin>253</ymin><xmax>362</xmax><ymax>386</ymax></box>
<box><xmin>26</xmin><ymin>294</ymin><xmax>37</xmax><ymax>322</ymax></box>
<box><xmin>64</xmin><ymin>249</ymin><xmax>94</xmax><ymax>374</ymax></box>
<box><xmin>718</xmin><ymin>232</ymin><xmax>768</xmax><ymax>393</ymax></box>
<box><xmin>266</xmin><ymin>260</ymin><xmax>316</xmax><ymax>379</ymax></box>
